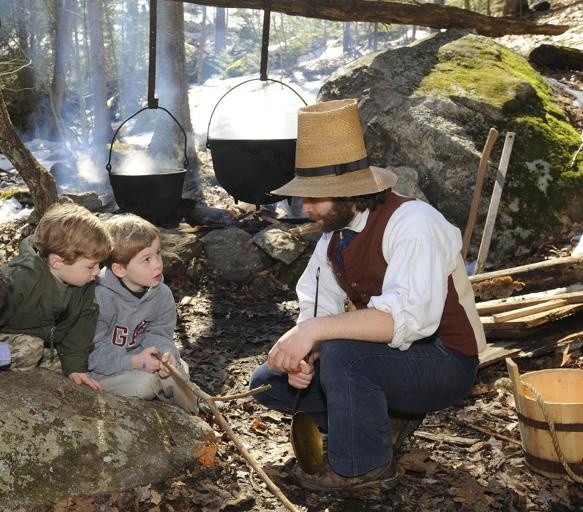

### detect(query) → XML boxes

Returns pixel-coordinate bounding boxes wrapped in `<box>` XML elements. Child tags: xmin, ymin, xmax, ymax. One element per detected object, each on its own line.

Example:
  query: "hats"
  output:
<box><xmin>270</xmin><ymin>99</ymin><xmax>398</xmax><ymax>198</ymax></box>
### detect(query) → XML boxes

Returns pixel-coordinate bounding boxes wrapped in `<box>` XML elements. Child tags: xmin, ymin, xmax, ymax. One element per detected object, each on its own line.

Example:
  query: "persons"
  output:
<box><xmin>243</xmin><ymin>96</ymin><xmax>489</xmax><ymax>494</ymax></box>
<box><xmin>86</xmin><ymin>211</ymin><xmax>201</xmax><ymax>418</ymax></box>
<box><xmin>0</xmin><ymin>200</ymin><xmax>115</xmax><ymax>393</ymax></box>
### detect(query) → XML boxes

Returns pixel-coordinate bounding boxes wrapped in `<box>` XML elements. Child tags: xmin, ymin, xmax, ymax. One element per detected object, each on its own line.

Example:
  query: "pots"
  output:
<box><xmin>206</xmin><ymin>77</ymin><xmax>308</xmax><ymax>207</ymax></box>
<box><xmin>108</xmin><ymin>106</ymin><xmax>187</xmax><ymax>222</ymax></box>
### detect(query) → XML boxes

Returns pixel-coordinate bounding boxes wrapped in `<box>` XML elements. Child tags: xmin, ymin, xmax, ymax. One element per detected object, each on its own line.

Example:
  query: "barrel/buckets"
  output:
<box><xmin>502</xmin><ymin>355</ymin><xmax>583</xmax><ymax>481</ymax></box>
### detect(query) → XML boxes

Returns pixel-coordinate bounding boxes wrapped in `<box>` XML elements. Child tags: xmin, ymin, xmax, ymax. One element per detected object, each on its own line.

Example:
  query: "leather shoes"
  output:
<box><xmin>302</xmin><ymin>463</ymin><xmax>399</xmax><ymax>493</ymax></box>
